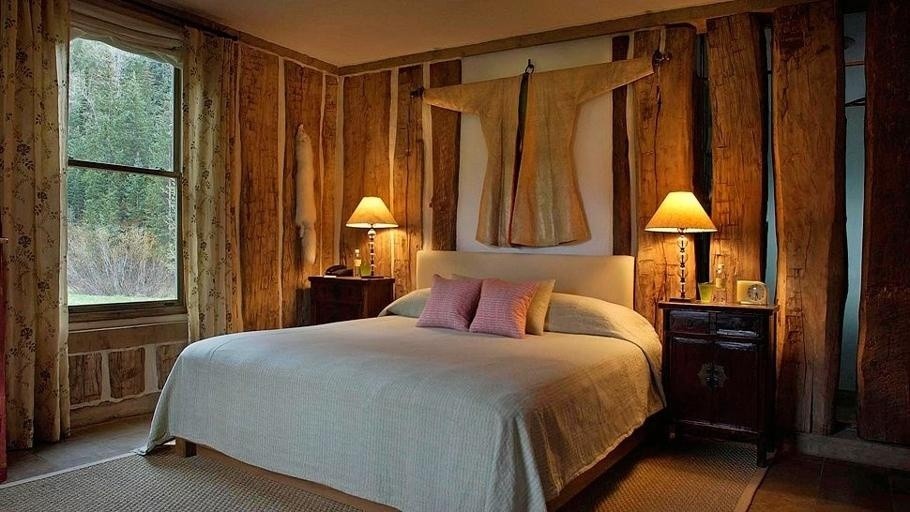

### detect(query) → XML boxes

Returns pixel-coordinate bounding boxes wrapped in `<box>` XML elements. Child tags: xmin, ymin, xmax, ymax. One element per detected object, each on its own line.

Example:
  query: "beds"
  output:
<box><xmin>174</xmin><ymin>252</ymin><xmax>641</xmax><ymax>512</ymax></box>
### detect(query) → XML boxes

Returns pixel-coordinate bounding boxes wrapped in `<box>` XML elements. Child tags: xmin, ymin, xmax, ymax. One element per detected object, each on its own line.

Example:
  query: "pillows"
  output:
<box><xmin>414</xmin><ymin>272</ymin><xmax>556</xmax><ymax>338</ymax></box>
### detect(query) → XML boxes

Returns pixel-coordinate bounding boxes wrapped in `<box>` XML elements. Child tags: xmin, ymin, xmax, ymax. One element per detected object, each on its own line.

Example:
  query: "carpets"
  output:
<box><xmin>0</xmin><ymin>441</ymin><xmax>771</xmax><ymax>511</ymax></box>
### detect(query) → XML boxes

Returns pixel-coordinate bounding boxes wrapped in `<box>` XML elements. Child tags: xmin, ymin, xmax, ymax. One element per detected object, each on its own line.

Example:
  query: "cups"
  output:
<box><xmin>699</xmin><ymin>283</ymin><xmax>715</xmax><ymax>302</ymax></box>
<box><xmin>360</xmin><ymin>263</ymin><xmax>371</xmax><ymax>276</ymax></box>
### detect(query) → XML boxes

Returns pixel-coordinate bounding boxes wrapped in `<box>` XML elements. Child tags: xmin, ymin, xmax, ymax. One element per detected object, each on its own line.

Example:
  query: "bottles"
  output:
<box><xmin>353</xmin><ymin>249</ymin><xmax>362</xmax><ymax>276</ymax></box>
<box><xmin>716</xmin><ymin>264</ymin><xmax>728</xmax><ymax>303</ymax></box>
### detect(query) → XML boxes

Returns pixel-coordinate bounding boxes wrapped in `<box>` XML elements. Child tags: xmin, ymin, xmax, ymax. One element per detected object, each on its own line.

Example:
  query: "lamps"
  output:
<box><xmin>644</xmin><ymin>191</ymin><xmax>718</xmax><ymax>297</ymax></box>
<box><xmin>345</xmin><ymin>195</ymin><xmax>400</xmax><ymax>278</ymax></box>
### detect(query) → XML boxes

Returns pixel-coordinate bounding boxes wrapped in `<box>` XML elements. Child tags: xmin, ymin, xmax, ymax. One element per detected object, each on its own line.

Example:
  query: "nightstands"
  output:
<box><xmin>659</xmin><ymin>299</ymin><xmax>776</xmax><ymax>464</ymax></box>
<box><xmin>308</xmin><ymin>275</ymin><xmax>395</xmax><ymax>321</ymax></box>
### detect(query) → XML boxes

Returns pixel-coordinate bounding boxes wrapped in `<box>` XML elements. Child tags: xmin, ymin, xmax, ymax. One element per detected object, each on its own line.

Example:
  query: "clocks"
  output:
<box><xmin>738</xmin><ymin>280</ymin><xmax>768</xmax><ymax>304</ymax></box>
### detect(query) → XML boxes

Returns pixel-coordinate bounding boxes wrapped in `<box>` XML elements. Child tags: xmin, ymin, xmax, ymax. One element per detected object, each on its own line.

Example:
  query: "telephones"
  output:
<box><xmin>325</xmin><ymin>264</ymin><xmax>353</xmax><ymax>277</ymax></box>
